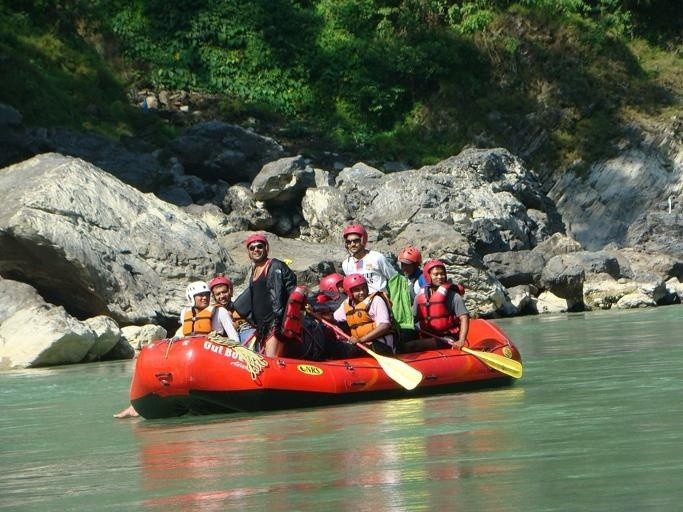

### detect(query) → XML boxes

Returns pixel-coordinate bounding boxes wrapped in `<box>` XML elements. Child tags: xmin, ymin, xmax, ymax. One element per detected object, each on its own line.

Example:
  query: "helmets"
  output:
<box><xmin>343</xmin><ymin>224</ymin><xmax>368</xmax><ymax>246</ymax></box>
<box><xmin>247</xmin><ymin>235</ymin><xmax>269</xmax><ymax>255</ymax></box>
<box><xmin>343</xmin><ymin>274</ymin><xmax>369</xmax><ymax>299</ymax></box>
<box><xmin>320</xmin><ymin>273</ymin><xmax>344</xmax><ymax>300</ymax></box>
<box><xmin>208</xmin><ymin>278</ymin><xmax>233</xmax><ymax>297</ymax></box>
<box><xmin>186</xmin><ymin>281</ymin><xmax>211</xmax><ymax>307</ymax></box>
<box><xmin>423</xmin><ymin>261</ymin><xmax>447</xmax><ymax>284</ymax></box>
<box><xmin>398</xmin><ymin>247</ymin><xmax>422</xmax><ymax>267</ymax></box>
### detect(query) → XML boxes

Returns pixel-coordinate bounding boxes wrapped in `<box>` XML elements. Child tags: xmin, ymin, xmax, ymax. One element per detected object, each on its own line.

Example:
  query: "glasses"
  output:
<box><xmin>345</xmin><ymin>238</ymin><xmax>362</xmax><ymax>244</ymax></box>
<box><xmin>248</xmin><ymin>244</ymin><xmax>265</xmax><ymax>252</ymax></box>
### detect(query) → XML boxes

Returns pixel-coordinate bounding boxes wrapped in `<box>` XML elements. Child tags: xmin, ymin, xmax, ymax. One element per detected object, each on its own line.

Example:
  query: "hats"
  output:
<box><xmin>399</xmin><ymin>259</ymin><xmax>412</xmax><ymax>265</ymax></box>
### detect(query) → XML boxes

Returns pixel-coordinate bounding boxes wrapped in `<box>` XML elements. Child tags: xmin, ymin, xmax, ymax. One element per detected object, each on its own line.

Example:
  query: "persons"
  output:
<box><xmin>320</xmin><ymin>273</ymin><xmax>343</xmax><ymax>304</ymax></box>
<box><xmin>232</xmin><ymin>234</ymin><xmax>305</xmax><ymax>358</ymax></box>
<box><xmin>210</xmin><ymin>277</ymin><xmax>235</xmax><ymax>310</ymax></box>
<box><xmin>343</xmin><ymin>225</ymin><xmax>412</xmax><ymax>355</ymax></box>
<box><xmin>411</xmin><ymin>261</ymin><xmax>468</xmax><ymax>350</ymax></box>
<box><xmin>399</xmin><ymin>246</ymin><xmax>422</xmax><ymax>279</ymax></box>
<box><xmin>305</xmin><ymin>275</ymin><xmax>394</xmax><ymax>357</ymax></box>
<box><xmin>180</xmin><ymin>281</ymin><xmax>239</xmax><ymax>347</ymax></box>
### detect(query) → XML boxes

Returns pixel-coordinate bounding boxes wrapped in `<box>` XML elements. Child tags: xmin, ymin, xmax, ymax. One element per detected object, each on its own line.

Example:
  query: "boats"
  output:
<box><xmin>129</xmin><ymin>319</ymin><xmax>522</xmax><ymax>420</ymax></box>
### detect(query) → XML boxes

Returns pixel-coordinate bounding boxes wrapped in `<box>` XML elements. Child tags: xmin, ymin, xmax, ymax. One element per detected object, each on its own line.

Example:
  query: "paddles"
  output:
<box><xmin>307</xmin><ymin>309</ymin><xmax>422</xmax><ymax>390</ymax></box>
<box><xmin>240</xmin><ymin>331</ymin><xmax>255</xmax><ymax>346</ymax></box>
<box><xmin>415</xmin><ymin>328</ymin><xmax>522</xmax><ymax>379</ymax></box>
<box><xmin>258</xmin><ymin>346</ymin><xmax>264</xmax><ymax>355</ymax></box>
